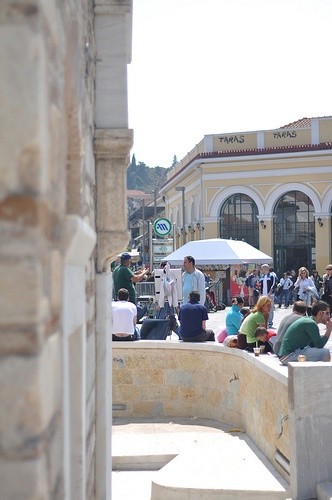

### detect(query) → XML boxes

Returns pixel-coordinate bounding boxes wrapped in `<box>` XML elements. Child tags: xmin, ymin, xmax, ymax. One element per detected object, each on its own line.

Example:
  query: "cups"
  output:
<box><xmin>253</xmin><ymin>348</ymin><xmax>260</xmax><ymax>356</ymax></box>
<box><xmin>297</xmin><ymin>355</ymin><xmax>306</xmax><ymax>362</ymax></box>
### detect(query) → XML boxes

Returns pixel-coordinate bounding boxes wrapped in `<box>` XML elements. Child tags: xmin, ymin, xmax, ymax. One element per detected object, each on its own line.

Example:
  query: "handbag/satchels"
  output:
<box><xmin>278</xmin><ymin>286</ymin><xmax>283</xmax><ymax>293</ymax></box>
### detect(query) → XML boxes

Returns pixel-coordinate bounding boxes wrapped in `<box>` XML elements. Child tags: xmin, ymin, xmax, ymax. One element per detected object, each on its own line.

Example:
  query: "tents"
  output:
<box><xmin>161</xmin><ymin>239</ymin><xmax>273</xmax><ymax>270</ymax></box>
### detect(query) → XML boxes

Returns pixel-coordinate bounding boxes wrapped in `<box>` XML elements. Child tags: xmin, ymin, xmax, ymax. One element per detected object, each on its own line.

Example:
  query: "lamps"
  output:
<box><xmin>169</xmin><ymin>223</ymin><xmax>204</xmax><ymax>238</ymax></box>
<box><xmin>318</xmin><ymin>217</ymin><xmax>323</xmax><ymax>227</ymax></box>
<box><xmin>260</xmin><ymin>220</ymin><xmax>266</xmax><ymax>229</ymax></box>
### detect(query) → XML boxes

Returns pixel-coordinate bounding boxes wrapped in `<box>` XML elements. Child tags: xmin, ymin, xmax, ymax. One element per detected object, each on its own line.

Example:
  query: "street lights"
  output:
<box><xmin>174</xmin><ymin>186</ymin><xmax>187</xmax><ymax>245</ymax></box>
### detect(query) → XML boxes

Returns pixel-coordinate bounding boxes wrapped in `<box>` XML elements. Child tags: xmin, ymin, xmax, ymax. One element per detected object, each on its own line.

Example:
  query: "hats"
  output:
<box><xmin>120</xmin><ymin>253</ymin><xmax>133</xmax><ymax>260</ymax></box>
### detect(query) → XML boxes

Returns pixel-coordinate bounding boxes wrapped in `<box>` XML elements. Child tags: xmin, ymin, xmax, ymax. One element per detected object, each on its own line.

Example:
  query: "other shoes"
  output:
<box><xmin>285</xmin><ymin>306</ymin><xmax>287</xmax><ymax>309</ymax></box>
<box><xmin>279</xmin><ymin>305</ymin><xmax>281</xmax><ymax>308</ymax></box>
<box><xmin>269</xmin><ymin>324</ymin><xmax>272</xmax><ymax>328</ymax></box>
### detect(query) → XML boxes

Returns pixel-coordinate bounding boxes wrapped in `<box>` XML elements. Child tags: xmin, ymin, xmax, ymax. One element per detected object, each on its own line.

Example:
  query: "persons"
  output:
<box><xmin>112</xmin><ymin>288</ymin><xmax>140</xmax><ymax>341</ymax></box>
<box><xmin>236</xmin><ymin>296</ymin><xmax>272</xmax><ymax>351</ymax></box>
<box><xmin>182</xmin><ymin>256</ymin><xmax>206</xmax><ymax>305</ymax></box>
<box><xmin>276</xmin><ymin>300</ymin><xmax>332</xmax><ymax>366</ymax></box>
<box><xmin>201</xmin><ymin>262</ymin><xmax>332</xmax><ymax>317</ymax></box>
<box><xmin>218</xmin><ymin>307</ymin><xmax>251</xmax><ymax>343</ymax></box>
<box><xmin>226</xmin><ymin>296</ymin><xmax>244</xmax><ymax>336</ymax></box>
<box><xmin>273</xmin><ymin>300</ymin><xmax>307</xmax><ymax>357</ymax></box>
<box><xmin>112</xmin><ymin>252</ymin><xmax>152</xmax><ymax>325</ymax></box>
<box><xmin>177</xmin><ymin>290</ymin><xmax>215</xmax><ymax>342</ymax></box>
<box><xmin>254</xmin><ymin>327</ymin><xmax>277</xmax><ymax>352</ymax></box>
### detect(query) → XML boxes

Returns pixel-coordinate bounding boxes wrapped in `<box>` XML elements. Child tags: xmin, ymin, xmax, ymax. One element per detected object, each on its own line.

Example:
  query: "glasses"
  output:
<box><xmin>326</xmin><ymin>269</ymin><xmax>332</xmax><ymax>270</ymax></box>
